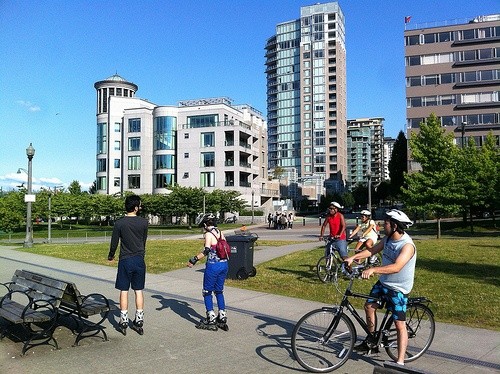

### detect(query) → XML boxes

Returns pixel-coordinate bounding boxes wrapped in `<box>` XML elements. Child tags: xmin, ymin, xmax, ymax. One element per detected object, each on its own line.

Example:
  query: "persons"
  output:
<box><xmin>186</xmin><ymin>213</ymin><xmax>229</xmax><ymax>332</ymax></box>
<box><xmin>343</xmin><ymin>209</ymin><xmax>417</xmax><ymax>366</ymax></box>
<box><xmin>349</xmin><ymin>210</ymin><xmax>381</xmax><ymax>279</ymax></box>
<box><xmin>319</xmin><ymin>202</ymin><xmax>352</xmax><ymax>280</ymax></box>
<box><xmin>107</xmin><ymin>194</ymin><xmax>148</xmax><ymax>336</ymax></box>
<box><xmin>267</xmin><ymin>212</ymin><xmax>294</xmax><ymax>230</ymax></box>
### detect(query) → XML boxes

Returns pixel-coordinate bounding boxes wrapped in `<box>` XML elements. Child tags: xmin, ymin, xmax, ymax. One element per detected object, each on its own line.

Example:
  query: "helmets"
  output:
<box><xmin>330</xmin><ymin>201</ymin><xmax>343</xmax><ymax>209</ymax></box>
<box><xmin>198</xmin><ymin>213</ymin><xmax>215</xmax><ymax>228</ymax></box>
<box><xmin>360</xmin><ymin>209</ymin><xmax>371</xmax><ymax>216</ymax></box>
<box><xmin>384</xmin><ymin>208</ymin><xmax>413</xmax><ymax>230</ymax></box>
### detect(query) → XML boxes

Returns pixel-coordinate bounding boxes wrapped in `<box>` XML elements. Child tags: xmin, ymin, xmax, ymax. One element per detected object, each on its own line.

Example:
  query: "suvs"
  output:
<box><xmin>371</xmin><ymin>204</ymin><xmax>377</xmax><ymax>210</ymax></box>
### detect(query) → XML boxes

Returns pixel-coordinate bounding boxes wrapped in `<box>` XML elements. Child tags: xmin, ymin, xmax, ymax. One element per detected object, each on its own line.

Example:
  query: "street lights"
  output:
<box><xmin>461</xmin><ymin>122</ymin><xmax>468</xmax><ymax>228</ymax></box>
<box><xmin>47</xmin><ymin>188</ymin><xmax>52</xmax><ymax>243</ymax></box>
<box><xmin>251</xmin><ymin>190</ymin><xmax>255</xmax><ymax>224</ymax></box>
<box><xmin>202</xmin><ymin>188</ymin><xmax>206</xmax><ymax>214</ymax></box>
<box><xmin>17</xmin><ymin>143</ymin><xmax>36</xmax><ymax>248</ymax></box>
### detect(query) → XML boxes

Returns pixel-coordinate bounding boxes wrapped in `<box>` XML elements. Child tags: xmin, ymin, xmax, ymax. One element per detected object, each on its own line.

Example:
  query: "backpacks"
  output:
<box><xmin>209</xmin><ymin>229</ymin><xmax>231</xmax><ymax>259</ymax></box>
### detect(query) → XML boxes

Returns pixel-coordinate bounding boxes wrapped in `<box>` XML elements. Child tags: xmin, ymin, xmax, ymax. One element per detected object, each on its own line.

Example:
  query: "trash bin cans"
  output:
<box><xmin>225</xmin><ymin>236</ymin><xmax>260</xmax><ymax>280</ymax></box>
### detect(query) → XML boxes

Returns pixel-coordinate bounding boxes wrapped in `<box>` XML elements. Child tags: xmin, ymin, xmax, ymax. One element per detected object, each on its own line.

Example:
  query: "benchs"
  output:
<box><xmin>22</xmin><ymin>269</ymin><xmax>117</xmax><ymax>348</ymax></box>
<box><xmin>0</xmin><ymin>269</ymin><xmax>68</xmax><ymax>356</ymax></box>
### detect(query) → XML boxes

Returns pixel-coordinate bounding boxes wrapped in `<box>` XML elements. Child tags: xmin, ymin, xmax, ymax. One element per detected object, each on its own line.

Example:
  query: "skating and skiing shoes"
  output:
<box><xmin>118</xmin><ymin>309</ymin><xmax>130</xmax><ymax>337</ymax></box>
<box><xmin>132</xmin><ymin>309</ymin><xmax>146</xmax><ymax>335</ymax></box>
<box><xmin>196</xmin><ymin>310</ymin><xmax>218</xmax><ymax>331</ymax></box>
<box><xmin>216</xmin><ymin>309</ymin><xmax>229</xmax><ymax>331</ymax></box>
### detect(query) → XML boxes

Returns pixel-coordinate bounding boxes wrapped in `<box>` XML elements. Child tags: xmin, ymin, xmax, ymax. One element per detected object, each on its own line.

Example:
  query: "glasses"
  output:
<box><xmin>329</xmin><ymin>207</ymin><xmax>335</xmax><ymax>210</ymax></box>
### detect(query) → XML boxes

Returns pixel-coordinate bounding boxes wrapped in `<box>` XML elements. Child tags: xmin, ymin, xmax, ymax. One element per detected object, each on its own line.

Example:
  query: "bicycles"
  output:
<box><xmin>316</xmin><ymin>236</ymin><xmax>382</xmax><ymax>284</ymax></box>
<box><xmin>290</xmin><ymin>261</ymin><xmax>435</xmax><ymax>373</ymax></box>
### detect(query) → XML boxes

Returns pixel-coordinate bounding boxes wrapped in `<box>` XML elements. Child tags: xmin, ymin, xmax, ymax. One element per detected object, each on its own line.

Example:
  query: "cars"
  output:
<box><xmin>342</xmin><ymin>209</ymin><xmax>365</xmax><ymax>214</ymax></box>
<box><xmin>319</xmin><ymin>209</ymin><xmax>331</xmax><ymax>218</ymax></box>
<box><xmin>380</xmin><ymin>206</ymin><xmax>402</xmax><ymax>212</ymax></box>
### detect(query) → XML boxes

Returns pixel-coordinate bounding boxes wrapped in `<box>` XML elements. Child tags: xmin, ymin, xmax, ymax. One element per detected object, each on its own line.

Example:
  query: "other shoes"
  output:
<box><xmin>352</xmin><ymin>340</ymin><xmax>381</xmax><ymax>355</ymax></box>
<box><xmin>369</xmin><ymin>255</ymin><xmax>377</xmax><ymax>264</ymax></box>
